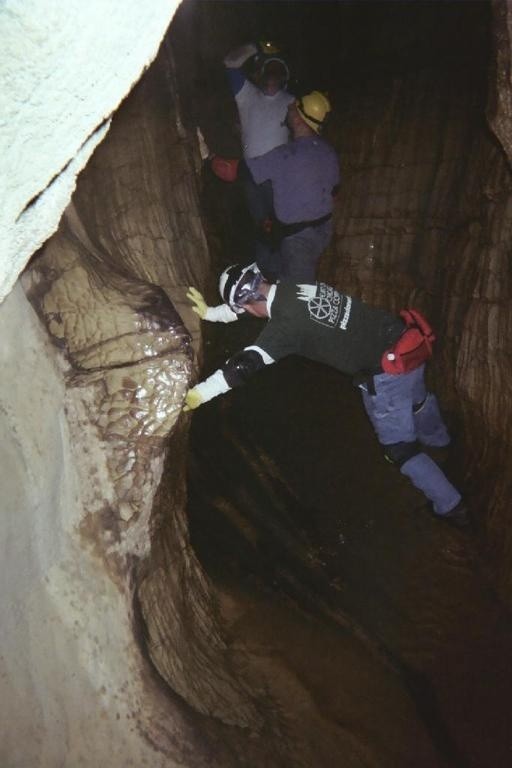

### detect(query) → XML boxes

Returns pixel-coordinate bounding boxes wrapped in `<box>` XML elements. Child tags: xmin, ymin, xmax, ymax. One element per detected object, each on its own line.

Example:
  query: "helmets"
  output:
<box><xmin>262</xmin><ymin>55</ymin><xmax>290</xmax><ymax>91</ymax></box>
<box><xmin>296</xmin><ymin>90</ymin><xmax>330</xmax><ymax>135</ymax></box>
<box><xmin>219</xmin><ymin>262</ymin><xmax>266</xmax><ymax>314</ymax></box>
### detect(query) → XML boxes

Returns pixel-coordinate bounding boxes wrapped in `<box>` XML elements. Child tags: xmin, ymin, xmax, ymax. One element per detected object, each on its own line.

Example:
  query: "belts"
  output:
<box><xmin>270</xmin><ymin>213</ymin><xmax>331</xmax><ymax>231</ymax></box>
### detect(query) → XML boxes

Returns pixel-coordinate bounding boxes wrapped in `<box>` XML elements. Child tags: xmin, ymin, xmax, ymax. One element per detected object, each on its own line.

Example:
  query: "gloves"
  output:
<box><xmin>183</xmin><ymin>389</ymin><xmax>201</xmax><ymax>412</ymax></box>
<box><xmin>186</xmin><ymin>287</ymin><xmax>208</xmax><ymax>320</ymax></box>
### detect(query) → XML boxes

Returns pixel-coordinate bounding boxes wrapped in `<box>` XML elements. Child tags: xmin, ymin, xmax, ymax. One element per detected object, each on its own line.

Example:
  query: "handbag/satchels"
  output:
<box><xmin>382</xmin><ymin>309</ymin><xmax>435</xmax><ymax>374</ymax></box>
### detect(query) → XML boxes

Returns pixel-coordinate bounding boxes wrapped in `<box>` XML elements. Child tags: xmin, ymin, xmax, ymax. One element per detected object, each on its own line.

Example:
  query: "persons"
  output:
<box><xmin>196</xmin><ymin>37</ymin><xmax>343</xmax><ymax>282</ymax></box>
<box><xmin>182</xmin><ymin>260</ymin><xmax>472</xmax><ymax>529</ymax></box>
